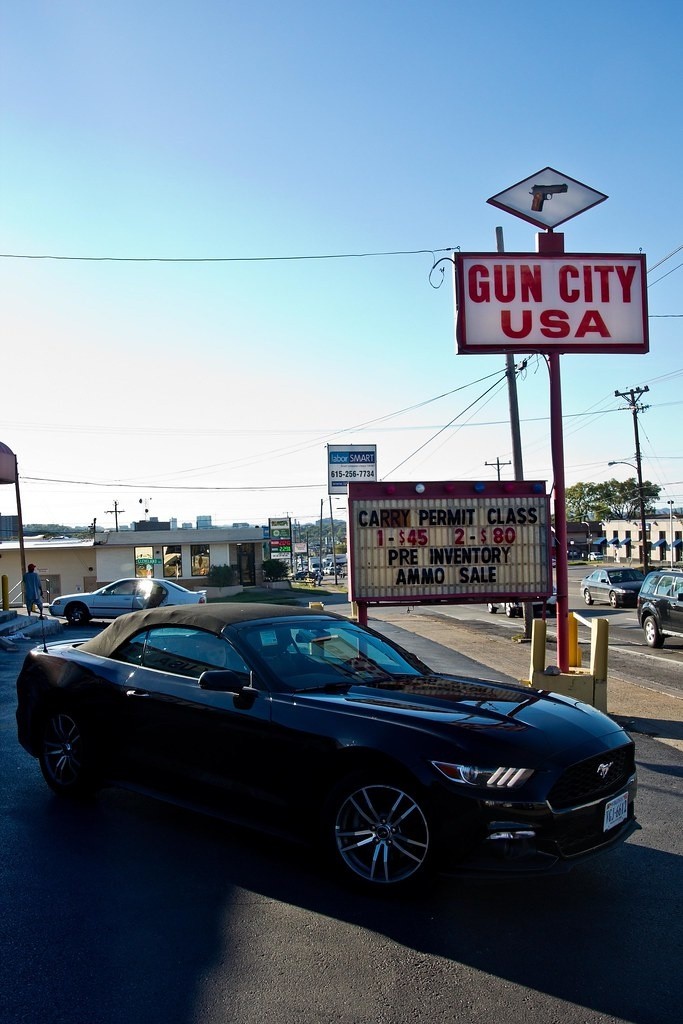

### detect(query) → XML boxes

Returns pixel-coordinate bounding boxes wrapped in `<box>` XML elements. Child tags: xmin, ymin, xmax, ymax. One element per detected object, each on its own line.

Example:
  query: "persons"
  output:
<box><xmin>198</xmin><ymin>635</ymin><xmax>226</xmax><ymax>670</ymax></box>
<box><xmin>149</xmin><ymin>587</ymin><xmax>165</xmax><ymax>608</ymax></box>
<box><xmin>23</xmin><ymin>564</ymin><xmax>45</xmax><ymax>620</ymax></box>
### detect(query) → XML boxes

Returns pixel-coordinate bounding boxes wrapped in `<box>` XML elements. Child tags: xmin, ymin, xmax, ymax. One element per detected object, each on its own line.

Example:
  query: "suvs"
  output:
<box><xmin>636</xmin><ymin>566</ymin><xmax>683</xmax><ymax>649</ymax></box>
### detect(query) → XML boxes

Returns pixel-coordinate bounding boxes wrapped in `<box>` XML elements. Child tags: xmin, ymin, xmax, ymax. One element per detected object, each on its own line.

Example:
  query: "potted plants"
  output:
<box><xmin>192</xmin><ymin>562</ymin><xmax>243</xmax><ymax>599</ymax></box>
<box><xmin>262</xmin><ymin>559</ymin><xmax>292</xmax><ymax>590</ymax></box>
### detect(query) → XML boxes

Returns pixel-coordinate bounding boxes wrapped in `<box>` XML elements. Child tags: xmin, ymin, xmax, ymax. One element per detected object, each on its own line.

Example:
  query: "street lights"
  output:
<box><xmin>668</xmin><ymin>499</ymin><xmax>674</xmax><ymax>568</ymax></box>
<box><xmin>608</xmin><ymin>461</ymin><xmax>648</xmax><ymax>577</ymax></box>
<box><xmin>581</xmin><ymin>521</ymin><xmax>590</xmax><ymax>554</ymax></box>
<box><xmin>318</xmin><ymin>497</ymin><xmax>340</xmax><ymax>586</ymax></box>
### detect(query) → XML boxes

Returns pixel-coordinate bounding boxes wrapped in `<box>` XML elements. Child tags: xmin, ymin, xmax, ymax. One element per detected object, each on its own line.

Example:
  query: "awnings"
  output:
<box><xmin>620</xmin><ymin>538</ymin><xmax>631</xmax><ymax>545</ymax></box>
<box><xmin>607</xmin><ymin>537</ymin><xmax>618</xmax><ymax>544</ymax></box>
<box><xmin>593</xmin><ymin>537</ymin><xmax>606</xmax><ymax>544</ymax></box>
<box><xmin>654</xmin><ymin>539</ymin><xmax>666</xmax><ymax>547</ymax></box>
<box><xmin>673</xmin><ymin>539</ymin><xmax>682</xmax><ymax>547</ymax></box>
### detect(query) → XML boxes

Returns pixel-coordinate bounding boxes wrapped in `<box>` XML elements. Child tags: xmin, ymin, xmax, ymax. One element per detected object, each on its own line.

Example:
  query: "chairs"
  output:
<box><xmin>611</xmin><ymin>578</ymin><xmax>619</xmax><ymax>583</ymax></box>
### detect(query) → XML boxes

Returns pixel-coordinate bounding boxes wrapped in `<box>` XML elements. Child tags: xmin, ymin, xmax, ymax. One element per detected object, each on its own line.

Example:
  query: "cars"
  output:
<box><xmin>587</xmin><ymin>551</ymin><xmax>603</xmax><ymax>561</ymax></box>
<box><xmin>486</xmin><ymin>586</ymin><xmax>558</xmax><ymax>618</ymax></box>
<box><xmin>47</xmin><ymin>577</ymin><xmax>208</xmax><ymax>628</ymax></box>
<box><xmin>291</xmin><ymin>552</ymin><xmax>348</xmax><ymax>581</ymax></box>
<box><xmin>578</xmin><ymin>567</ymin><xmax>646</xmax><ymax>609</ymax></box>
<box><xmin>12</xmin><ymin>600</ymin><xmax>642</xmax><ymax>897</ymax></box>
